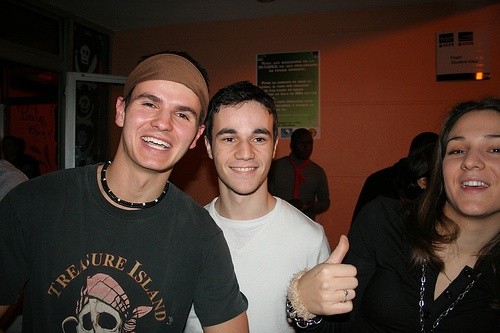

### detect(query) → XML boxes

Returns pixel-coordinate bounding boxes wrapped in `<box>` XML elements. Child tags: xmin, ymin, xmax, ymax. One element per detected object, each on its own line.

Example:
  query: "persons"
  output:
<box><xmin>184</xmin><ymin>80</ymin><xmax>331</xmax><ymax>333</ymax></box>
<box><xmin>267</xmin><ymin>129</ymin><xmax>330</xmax><ymax>220</ymax></box>
<box><xmin>0</xmin><ymin>53</ymin><xmax>250</xmax><ymax>333</ymax></box>
<box><xmin>350</xmin><ymin>132</ymin><xmax>439</xmax><ymax>230</ymax></box>
<box><xmin>285</xmin><ymin>97</ymin><xmax>500</xmax><ymax>333</ymax></box>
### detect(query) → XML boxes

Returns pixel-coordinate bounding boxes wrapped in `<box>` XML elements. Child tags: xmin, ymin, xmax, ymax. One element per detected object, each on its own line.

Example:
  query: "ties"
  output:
<box><xmin>288</xmin><ymin>159</ymin><xmax>311</xmax><ymax>200</ymax></box>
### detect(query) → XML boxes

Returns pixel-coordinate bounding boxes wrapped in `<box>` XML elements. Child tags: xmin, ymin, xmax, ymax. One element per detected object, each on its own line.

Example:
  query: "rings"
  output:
<box><xmin>340</xmin><ymin>290</ymin><xmax>348</xmax><ymax>302</ymax></box>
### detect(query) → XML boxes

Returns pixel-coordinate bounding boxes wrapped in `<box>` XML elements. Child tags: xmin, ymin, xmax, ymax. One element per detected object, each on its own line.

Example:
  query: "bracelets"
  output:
<box><xmin>286</xmin><ymin>268</ymin><xmax>322</xmax><ymax>328</ymax></box>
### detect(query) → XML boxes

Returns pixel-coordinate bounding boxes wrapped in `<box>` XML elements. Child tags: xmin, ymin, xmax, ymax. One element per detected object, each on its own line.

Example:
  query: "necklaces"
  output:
<box><xmin>416</xmin><ymin>259</ymin><xmax>475</xmax><ymax>333</ymax></box>
<box><xmin>101</xmin><ymin>160</ymin><xmax>169</xmax><ymax>208</ymax></box>
